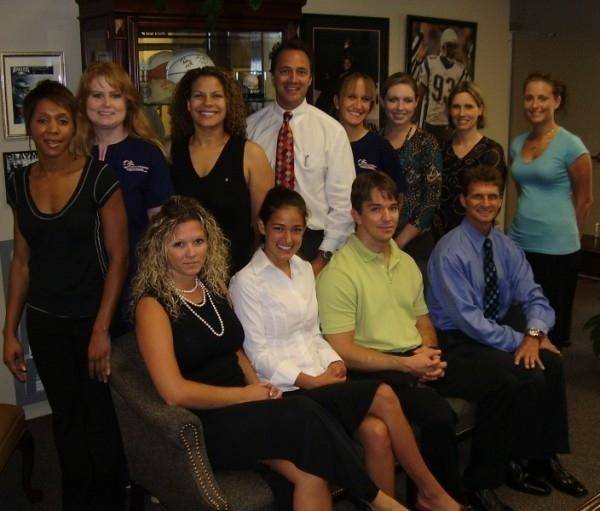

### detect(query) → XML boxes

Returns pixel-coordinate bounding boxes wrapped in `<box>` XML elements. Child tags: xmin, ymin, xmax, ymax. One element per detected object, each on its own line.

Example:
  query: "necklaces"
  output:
<box><xmin>163</xmin><ymin>273</ymin><xmax>228</xmax><ymax>337</ymax></box>
<box><xmin>523</xmin><ymin>125</ymin><xmax>555</xmax><ymax>152</ymax></box>
<box><xmin>378</xmin><ymin>122</ymin><xmax>417</xmax><ymax>148</ymax></box>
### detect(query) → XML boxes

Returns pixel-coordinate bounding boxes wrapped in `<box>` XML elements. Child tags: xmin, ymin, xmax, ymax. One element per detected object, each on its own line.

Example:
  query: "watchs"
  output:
<box><xmin>528</xmin><ymin>326</ymin><xmax>546</xmax><ymax>338</ymax></box>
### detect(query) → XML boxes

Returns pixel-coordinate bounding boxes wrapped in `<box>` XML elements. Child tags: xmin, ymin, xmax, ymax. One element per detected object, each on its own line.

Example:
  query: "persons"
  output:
<box><xmin>75</xmin><ymin>59</ymin><xmax>173</xmax><ymax>250</ymax></box>
<box><xmin>160</xmin><ymin>63</ymin><xmax>276</xmax><ymax>276</ymax></box>
<box><xmin>243</xmin><ymin>39</ymin><xmax>357</xmax><ymax>276</ymax></box>
<box><xmin>327</xmin><ymin>69</ymin><xmax>408</xmax><ymax>220</ymax></box>
<box><xmin>435</xmin><ymin>86</ymin><xmax>507</xmax><ymax>234</ymax></box>
<box><xmin>3</xmin><ymin>77</ymin><xmax>131</xmax><ymax>505</ymax></box>
<box><xmin>410</xmin><ymin>29</ymin><xmax>472</xmax><ymax>137</ymax></box>
<box><xmin>316</xmin><ymin>169</ymin><xmax>458</xmax><ymax>511</ymax></box>
<box><xmin>506</xmin><ymin>71</ymin><xmax>595</xmax><ymax>348</ymax></box>
<box><xmin>228</xmin><ymin>183</ymin><xmax>461</xmax><ymax>511</ymax></box>
<box><xmin>424</xmin><ymin>164</ymin><xmax>588</xmax><ymax>500</ymax></box>
<box><xmin>134</xmin><ymin>194</ymin><xmax>408</xmax><ymax>511</ymax></box>
<box><xmin>381</xmin><ymin>70</ymin><xmax>445</xmax><ymax>268</ymax></box>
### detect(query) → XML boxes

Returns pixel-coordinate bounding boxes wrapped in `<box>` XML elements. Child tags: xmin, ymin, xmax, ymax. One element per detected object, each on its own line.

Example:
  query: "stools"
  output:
<box><xmin>0</xmin><ymin>387</ymin><xmax>41</xmax><ymax>510</ymax></box>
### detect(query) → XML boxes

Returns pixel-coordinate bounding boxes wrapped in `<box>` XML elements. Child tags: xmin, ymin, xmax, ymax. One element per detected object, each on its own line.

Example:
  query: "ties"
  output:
<box><xmin>483</xmin><ymin>237</ymin><xmax>501</xmax><ymax>324</ymax></box>
<box><xmin>273</xmin><ymin>111</ymin><xmax>296</xmax><ymax>192</ymax></box>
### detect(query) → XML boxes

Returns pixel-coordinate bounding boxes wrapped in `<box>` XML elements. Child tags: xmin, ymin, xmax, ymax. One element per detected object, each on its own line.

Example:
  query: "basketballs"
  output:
<box><xmin>165</xmin><ymin>51</ymin><xmax>215</xmax><ymax>84</ymax></box>
<box><xmin>144</xmin><ymin>52</ymin><xmax>173</xmax><ymax>81</ymax></box>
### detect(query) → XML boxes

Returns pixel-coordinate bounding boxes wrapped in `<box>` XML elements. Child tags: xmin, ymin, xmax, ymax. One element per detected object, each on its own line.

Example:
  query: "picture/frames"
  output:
<box><xmin>305</xmin><ymin>14</ymin><xmax>390</xmax><ymax>128</ymax></box>
<box><xmin>408</xmin><ymin>15</ymin><xmax>478</xmax><ymax>136</ymax></box>
<box><xmin>3</xmin><ymin>54</ymin><xmax>67</xmax><ymax>141</ymax></box>
<box><xmin>4</xmin><ymin>150</ymin><xmax>48</xmax><ymax>181</ymax></box>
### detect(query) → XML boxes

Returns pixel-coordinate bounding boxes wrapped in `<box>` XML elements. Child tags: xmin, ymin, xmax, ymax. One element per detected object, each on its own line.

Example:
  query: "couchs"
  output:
<box><xmin>104</xmin><ymin>254</ymin><xmax>576</xmax><ymax>510</ymax></box>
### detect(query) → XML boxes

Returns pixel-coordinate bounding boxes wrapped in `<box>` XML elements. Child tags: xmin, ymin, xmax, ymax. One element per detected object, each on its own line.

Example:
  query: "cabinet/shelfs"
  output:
<box><xmin>76</xmin><ymin>2</ymin><xmax>308</xmax><ymax>152</ymax></box>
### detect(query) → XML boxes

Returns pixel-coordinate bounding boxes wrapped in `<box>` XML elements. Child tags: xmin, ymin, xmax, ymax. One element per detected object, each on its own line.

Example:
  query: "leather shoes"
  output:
<box><xmin>499</xmin><ymin>455</ymin><xmax>552</xmax><ymax>497</ymax></box>
<box><xmin>543</xmin><ymin>453</ymin><xmax>588</xmax><ymax>499</ymax></box>
